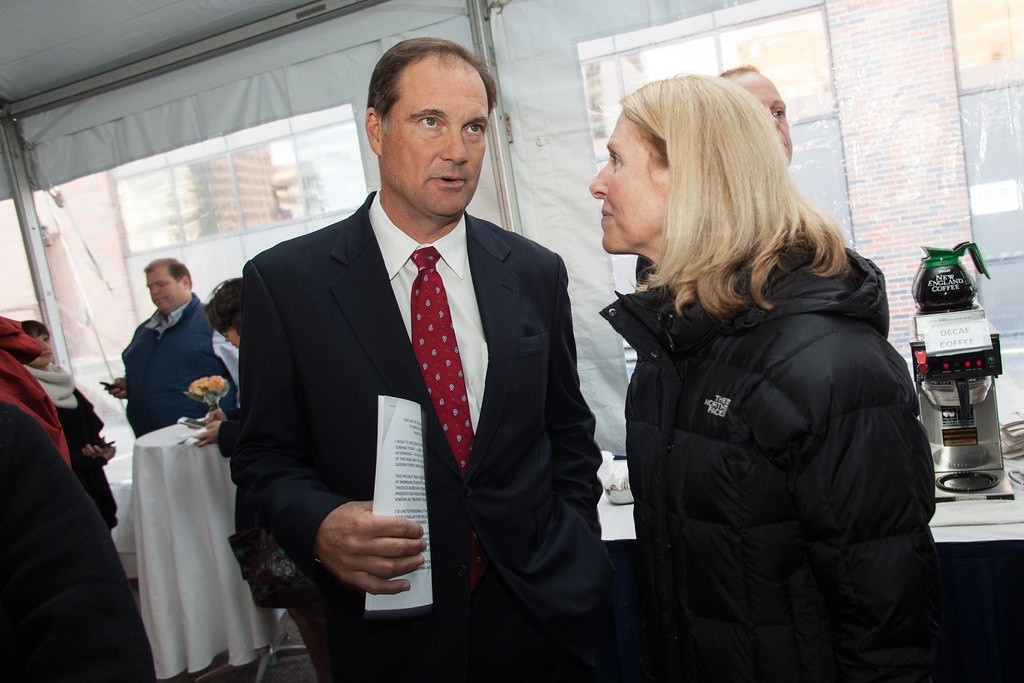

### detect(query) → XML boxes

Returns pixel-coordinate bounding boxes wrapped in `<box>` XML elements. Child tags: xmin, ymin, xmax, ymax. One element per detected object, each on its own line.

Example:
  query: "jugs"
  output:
<box><xmin>913</xmin><ymin>242</ymin><xmax>991</xmax><ymax>313</ymax></box>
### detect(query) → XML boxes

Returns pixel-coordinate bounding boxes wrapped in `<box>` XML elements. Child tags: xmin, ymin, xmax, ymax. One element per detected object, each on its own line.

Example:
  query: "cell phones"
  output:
<box><xmin>100</xmin><ymin>382</ymin><xmax>122</xmax><ymax>391</ymax></box>
<box><xmin>101</xmin><ymin>441</ymin><xmax>116</xmax><ymax>448</ymax></box>
<box><xmin>178</xmin><ymin>416</ymin><xmax>206</xmax><ymax>429</ymax></box>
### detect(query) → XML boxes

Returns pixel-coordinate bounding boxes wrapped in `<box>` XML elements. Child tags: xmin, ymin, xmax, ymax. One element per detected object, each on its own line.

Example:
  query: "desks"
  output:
<box><xmin>133</xmin><ymin>418</ymin><xmax>306</xmax><ymax>683</ymax></box>
<box><xmin>598</xmin><ymin>457</ymin><xmax>1024</xmax><ymax>683</ymax></box>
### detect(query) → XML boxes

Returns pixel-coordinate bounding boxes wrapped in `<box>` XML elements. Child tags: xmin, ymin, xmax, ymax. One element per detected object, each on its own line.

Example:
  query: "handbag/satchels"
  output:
<box><xmin>228</xmin><ymin>526</ymin><xmax>323</xmax><ymax>608</ymax></box>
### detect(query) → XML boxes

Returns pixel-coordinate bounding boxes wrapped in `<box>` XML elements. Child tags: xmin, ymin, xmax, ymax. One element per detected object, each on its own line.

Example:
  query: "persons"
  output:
<box><xmin>192</xmin><ymin>278</ymin><xmax>333</xmax><ymax>683</ymax></box>
<box><xmin>0</xmin><ymin>317</ymin><xmax>156</xmax><ymax>683</ymax></box>
<box><xmin>590</xmin><ymin>75</ymin><xmax>954</xmax><ymax>683</ymax></box>
<box><xmin>105</xmin><ymin>258</ymin><xmax>240</xmax><ymax>438</ymax></box>
<box><xmin>719</xmin><ymin>68</ymin><xmax>792</xmax><ymax>162</ymax></box>
<box><xmin>231</xmin><ymin>37</ymin><xmax>615</xmax><ymax>683</ymax></box>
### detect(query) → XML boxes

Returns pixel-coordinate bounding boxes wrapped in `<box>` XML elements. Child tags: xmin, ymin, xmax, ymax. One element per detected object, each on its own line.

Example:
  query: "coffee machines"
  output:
<box><xmin>909</xmin><ymin>315</ymin><xmax>1014</xmax><ymax>501</ymax></box>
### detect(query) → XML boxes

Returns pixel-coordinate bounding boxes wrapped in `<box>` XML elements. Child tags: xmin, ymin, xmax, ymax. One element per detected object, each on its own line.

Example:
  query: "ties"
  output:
<box><xmin>408</xmin><ymin>247</ymin><xmax>488</xmax><ymax>592</ymax></box>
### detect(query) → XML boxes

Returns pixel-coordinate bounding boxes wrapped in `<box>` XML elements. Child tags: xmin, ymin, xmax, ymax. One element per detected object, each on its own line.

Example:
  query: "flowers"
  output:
<box><xmin>183</xmin><ymin>375</ymin><xmax>229</xmax><ymax>411</ymax></box>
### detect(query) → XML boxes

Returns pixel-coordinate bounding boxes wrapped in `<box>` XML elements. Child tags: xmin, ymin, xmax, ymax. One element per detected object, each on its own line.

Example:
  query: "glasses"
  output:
<box><xmin>217</xmin><ymin>326</ymin><xmax>228</xmax><ymax>336</ymax></box>
